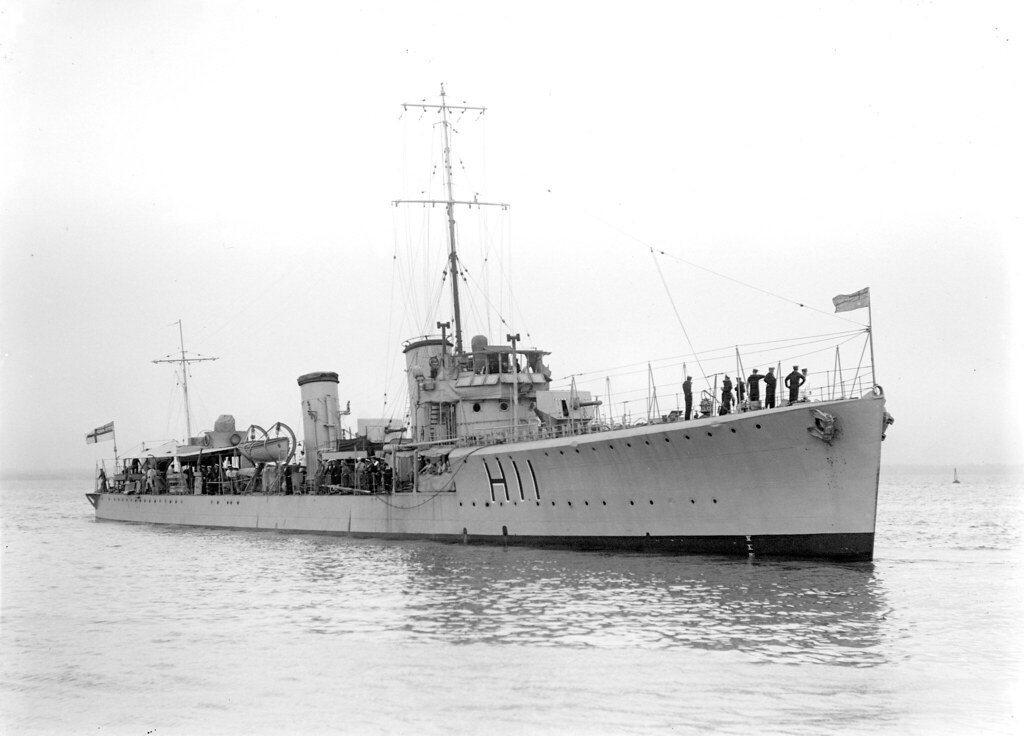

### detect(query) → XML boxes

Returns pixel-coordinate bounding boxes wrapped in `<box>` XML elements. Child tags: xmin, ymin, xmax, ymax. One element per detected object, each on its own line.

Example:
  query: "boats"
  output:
<box><xmin>81</xmin><ymin>81</ymin><xmax>893</xmax><ymax>566</ymax></box>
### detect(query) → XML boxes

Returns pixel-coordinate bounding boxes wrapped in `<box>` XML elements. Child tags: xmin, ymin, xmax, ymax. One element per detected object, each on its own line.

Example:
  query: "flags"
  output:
<box><xmin>832</xmin><ymin>287</ymin><xmax>869</xmax><ymax>313</ymax></box>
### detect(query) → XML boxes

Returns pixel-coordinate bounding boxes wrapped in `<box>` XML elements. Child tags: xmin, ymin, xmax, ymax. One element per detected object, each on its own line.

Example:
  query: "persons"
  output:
<box><xmin>746</xmin><ymin>368</ymin><xmax>766</xmax><ymax>410</ymax></box>
<box><xmin>682</xmin><ymin>376</ymin><xmax>693</xmax><ymax>421</ymax></box>
<box><xmin>96</xmin><ymin>456</ymin><xmax>452</xmax><ymax>495</ymax></box>
<box><xmin>722</xmin><ymin>391</ymin><xmax>735</xmax><ymax>414</ymax></box>
<box><xmin>735</xmin><ymin>378</ymin><xmax>747</xmax><ymax>406</ymax></box>
<box><xmin>785</xmin><ymin>365</ymin><xmax>806</xmax><ymax>407</ymax></box>
<box><xmin>720</xmin><ymin>374</ymin><xmax>733</xmax><ymax>392</ymax></box>
<box><xmin>764</xmin><ymin>366</ymin><xmax>777</xmax><ymax>408</ymax></box>
